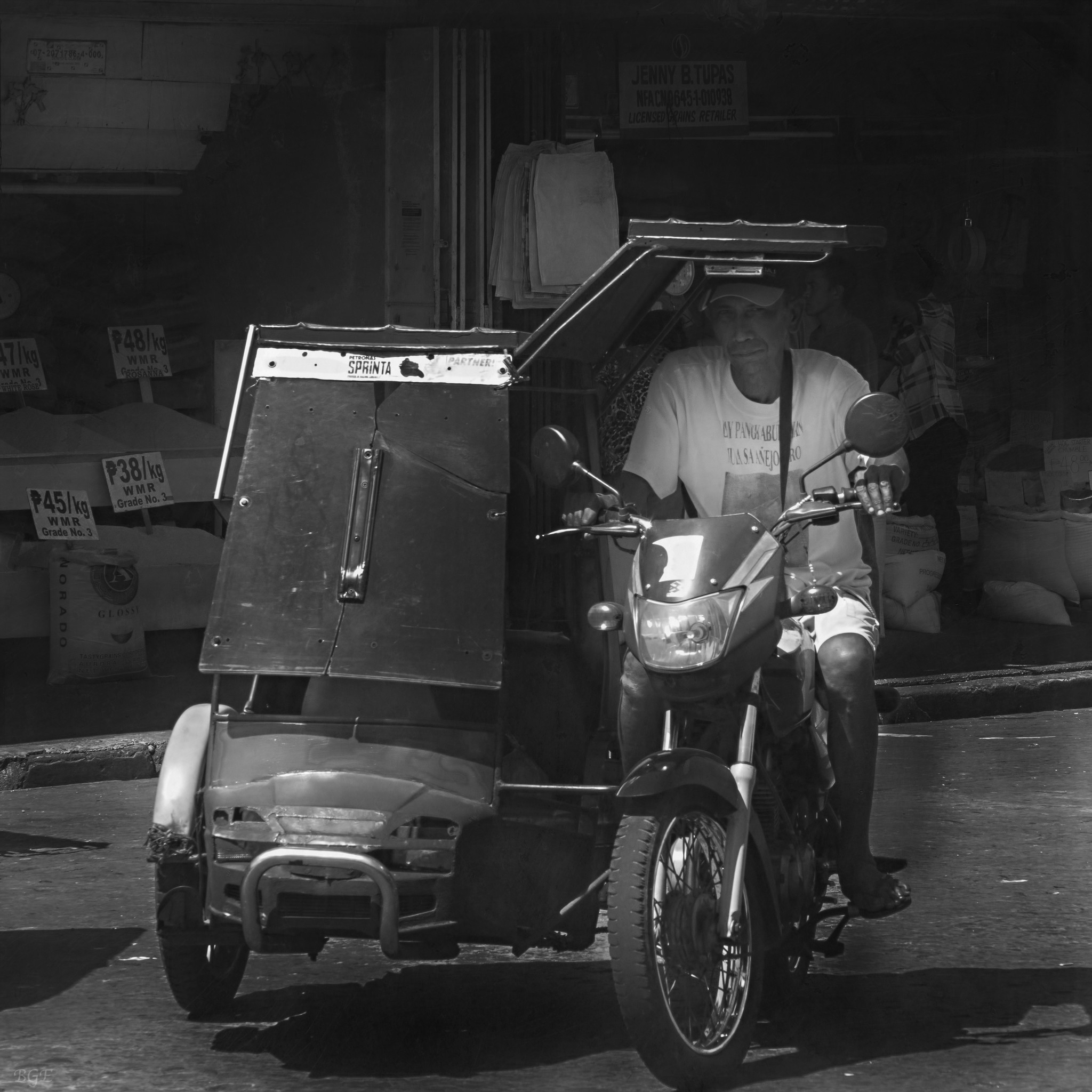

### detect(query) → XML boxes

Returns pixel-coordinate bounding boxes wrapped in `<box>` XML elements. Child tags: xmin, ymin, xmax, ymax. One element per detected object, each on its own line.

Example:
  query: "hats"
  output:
<box><xmin>709</xmin><ymin>267</ymin><xmax>785</xmax><ymax>309</ymax></box>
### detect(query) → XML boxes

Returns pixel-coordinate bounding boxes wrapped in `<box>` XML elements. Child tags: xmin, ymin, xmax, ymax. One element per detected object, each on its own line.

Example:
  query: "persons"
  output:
<box><xmin>592</xmin><ymin>309</ymin><xmax>689</xmax><ymax>472</ymax></box>
<box><xmin>556</xmin><ymin>271</ymin><xmax>912</xmax><ymax>919</ymax></box>
<box><xmin>804</xmin><ymin>255</ymin><xmax>878</xmax><ymax>392</ymax></box>
<box><xmin>877</xmin><ymin>249</ymin><xmax>967</xmax><ymax>621</ymax></box>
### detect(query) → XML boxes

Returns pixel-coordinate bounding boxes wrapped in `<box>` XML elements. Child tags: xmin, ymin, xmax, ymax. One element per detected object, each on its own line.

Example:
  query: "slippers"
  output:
<box><xmin>841</xmin><ymin>868</ymin><xmax>913</xmax><ymax>919</ymax></box>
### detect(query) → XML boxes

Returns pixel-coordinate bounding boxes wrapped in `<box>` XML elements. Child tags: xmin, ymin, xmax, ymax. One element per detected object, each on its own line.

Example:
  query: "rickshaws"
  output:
<box><xmin>144</xmin><ymin>216</ymin><xmax>912</xmax><ymax>1092</ymax></box>
